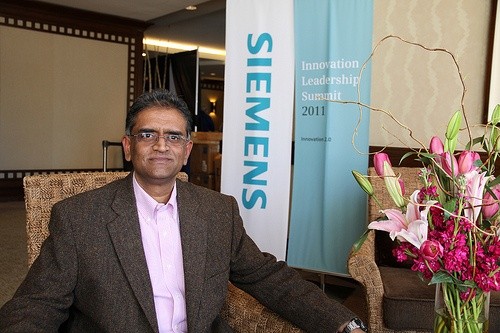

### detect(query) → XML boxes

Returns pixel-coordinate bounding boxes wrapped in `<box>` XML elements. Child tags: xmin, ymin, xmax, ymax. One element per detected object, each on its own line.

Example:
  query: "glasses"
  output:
<box><xmin>126</xmin><ymin>131</ymin><xmax>192</xmax><ymax>148</ymax></box>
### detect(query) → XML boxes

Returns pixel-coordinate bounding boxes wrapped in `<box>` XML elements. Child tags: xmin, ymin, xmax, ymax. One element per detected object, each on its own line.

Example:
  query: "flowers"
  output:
<box><xmin>316</xmin><ymin>34</ymin><xmax>500</xmax><ymax>333</ymax></box>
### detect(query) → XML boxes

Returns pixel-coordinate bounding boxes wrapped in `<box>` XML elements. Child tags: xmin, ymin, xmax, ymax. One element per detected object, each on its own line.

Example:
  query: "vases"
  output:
<box><xmin>435</xmin><ymin>282</ymin><xmax>486</xmax><ymax>333</ymax></box>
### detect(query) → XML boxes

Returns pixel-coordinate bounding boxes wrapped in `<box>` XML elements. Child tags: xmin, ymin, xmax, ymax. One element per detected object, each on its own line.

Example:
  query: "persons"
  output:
<box><xmin>0</xmin><ymin>89</ymin><xmax>369</xmax><ymax>333</ymax></box>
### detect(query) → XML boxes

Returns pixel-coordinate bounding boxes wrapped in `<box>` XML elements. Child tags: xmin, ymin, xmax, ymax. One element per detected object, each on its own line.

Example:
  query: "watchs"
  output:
<box><xmin>342</xmin><ymin>317</ymin><xmax>363</xmax><ymax>333</ymax></box>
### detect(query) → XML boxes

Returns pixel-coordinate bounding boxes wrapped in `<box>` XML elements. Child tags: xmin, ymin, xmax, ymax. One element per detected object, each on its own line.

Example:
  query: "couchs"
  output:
<box><xmin>347</xmin><ymin>166</ymin><xmax>500</xmax><ymax>333</ymax></box>
<box><xmin>22</xmin><ymin>170</ymin><xmax>302</xmax><ymax>332</ymax></box>
